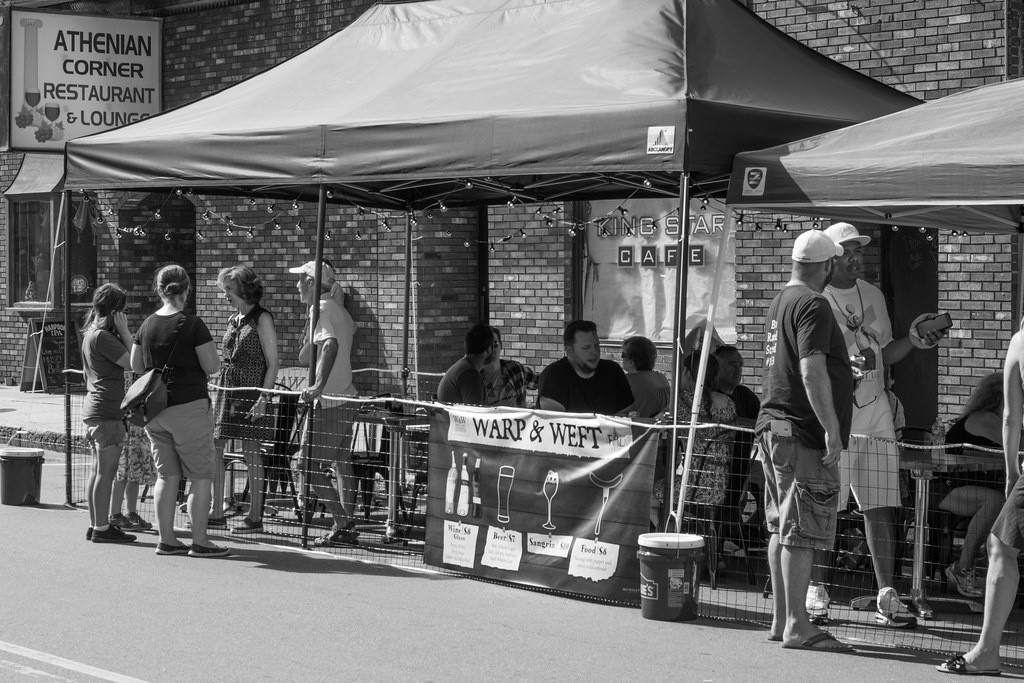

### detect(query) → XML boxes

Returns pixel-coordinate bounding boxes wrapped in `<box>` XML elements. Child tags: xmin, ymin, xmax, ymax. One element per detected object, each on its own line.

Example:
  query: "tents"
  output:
<box><xmin>670</xmin><ymin>80</ymin><xmax>1024</xmax><ymax>530</ymax></box>
<box><xmin>56</xmin><ymin>0</ymin><xmax>929</xmax><ymax>550</ymax></box>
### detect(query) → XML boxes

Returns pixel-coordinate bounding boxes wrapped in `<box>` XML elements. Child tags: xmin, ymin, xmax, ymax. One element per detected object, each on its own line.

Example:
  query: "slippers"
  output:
<box><xmin>936</xmin><ymin>654</ymin><xmax>1002</xmax><ymax>676</ymax></box>
<box><xmin>768</xmin><ymin>633</ymin><xmax>782</xmax><ymax>640</ymax></box>
<box><xmin>782</xmin><ymin>631</ymin><xmax>852</xmax><ymax>651</ymax></box>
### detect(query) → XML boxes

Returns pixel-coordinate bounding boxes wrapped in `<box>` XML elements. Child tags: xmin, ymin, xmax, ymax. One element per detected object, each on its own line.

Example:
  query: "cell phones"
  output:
<box><xmin>916</xmin><ymin>313</ymin><xmax>953</xmax><ymax>338</ymax></box>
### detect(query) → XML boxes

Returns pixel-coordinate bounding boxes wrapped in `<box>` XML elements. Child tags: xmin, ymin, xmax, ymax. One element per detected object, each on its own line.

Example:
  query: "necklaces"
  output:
<box><xmin>827</xmin><ymin>280</ymin><xmax>865</xmax><ymax>327</ymax></box>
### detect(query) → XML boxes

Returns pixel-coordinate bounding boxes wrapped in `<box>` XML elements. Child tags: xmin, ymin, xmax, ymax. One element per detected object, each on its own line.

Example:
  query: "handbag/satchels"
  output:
<box><xmin>120</xmin><ymin>368</ymin><xmax>167</xmax><ymax>428</ymax></box>
<box><xmin>229</xmin><ymin>402</ymin><xmax>254</xmax><ymax>417</ymax></box>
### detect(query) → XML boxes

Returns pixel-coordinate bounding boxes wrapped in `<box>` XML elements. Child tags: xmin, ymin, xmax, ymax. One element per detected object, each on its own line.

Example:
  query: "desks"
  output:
<box><xmin>357</xmin><ymin>413</ymin><xmax>429</xmax><ymax>538</ymax></box>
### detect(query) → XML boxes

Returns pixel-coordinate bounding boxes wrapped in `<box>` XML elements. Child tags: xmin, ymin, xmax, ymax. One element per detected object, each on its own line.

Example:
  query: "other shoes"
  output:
<box><xmin>314</xmin><ymin>521</ymin><xmax>359</xmax><ymax>547</ymax></box>
<box><xmin>91</xmin><ymin>523</ymin><xmax>136</xmax><ymax>543</ymax></box>
<box><xmin>945</xmin><ymin>560</ymin><xmax>984</xmax><ymax>598</ymax></box>
<box><xmin>109</xmin><ymin>515</ymin><xmax>139</xmax><ymax>530</ymax></box>
<box><xmin>231</xmin><ymin>517</ymin><xmax>264</xmax><ymax>533</ymax></box>
<box><xmin>186</xmin><ymin>516</ymin><xmax>227</xmax><ymax>529</ymax></box>
<box><xmin>188</xmin><ymin>543</ymin><xmax>229</xmax><ymax>557</ymax></box>
<box><xmin>125</xmin><ymin>513</ymin><xmax>152</xmax><ymax>529</ymax></box>
<box><xmin>155</xmin><ymin>542</ymin><xmax>190</xmax><ymax>554</ymax></box>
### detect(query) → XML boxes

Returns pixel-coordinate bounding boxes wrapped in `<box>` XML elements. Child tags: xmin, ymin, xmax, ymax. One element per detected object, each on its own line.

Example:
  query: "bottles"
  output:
<box><xmin>931</xmin><ymin>417</ymin><xmax>946</xmax><ymax>460</ymax></box>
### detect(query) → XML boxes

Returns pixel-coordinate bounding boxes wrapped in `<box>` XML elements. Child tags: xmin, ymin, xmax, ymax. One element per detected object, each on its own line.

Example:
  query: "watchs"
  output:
<box><xmin>259</xmin><ymin>393</ymin><xmax>270</xmax><ymax>401</ymax></box>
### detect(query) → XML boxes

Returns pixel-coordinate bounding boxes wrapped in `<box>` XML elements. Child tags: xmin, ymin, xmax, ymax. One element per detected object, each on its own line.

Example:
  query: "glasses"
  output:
<box><xmin>621</xmin><ymin>351</ymin><xmax>627</xmax><ymax>358</ymax></box>
<box><xmin>493</xmin><ymin>341</ymin><xmax>499</xmax><ymax>347</ymax></box>
<box><xmin>852</xmin><ymin>367</ymin><xmax>877</xmax><ymax>409</ymax></box>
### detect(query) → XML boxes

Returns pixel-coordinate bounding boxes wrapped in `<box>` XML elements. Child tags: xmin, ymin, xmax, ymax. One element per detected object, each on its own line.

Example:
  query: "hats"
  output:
<box><xmin>289</xmin><ymin>261</ymin><xmax>335</xmax><ymax>289</ymax></box>
<box><xmin>791</xmin><ymin>230</ymin><xmax>844</xmax><ymax>263</ymax></box>
<box><xmin>822</xmin><ymin>222</ymin><xmax>872</xmax><ymax>246</ymax></box>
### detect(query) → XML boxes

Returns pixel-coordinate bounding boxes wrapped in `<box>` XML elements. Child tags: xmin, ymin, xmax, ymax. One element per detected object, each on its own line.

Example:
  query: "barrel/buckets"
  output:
<box><xmin>0</xmin><ymin>431</ymin><xmax>46</xmax><ymax>505</ymax></box>
<box><xmin>636</xmin><ymin>511</ymin><xmax>705</xmax><ymax>620</ymax></box>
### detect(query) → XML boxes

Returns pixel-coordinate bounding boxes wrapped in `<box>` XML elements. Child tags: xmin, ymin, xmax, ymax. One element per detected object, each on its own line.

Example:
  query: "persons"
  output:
<box><xmin>80</xmin><ymin>221</ymin><xmax>1024</xmax><ymax>676</ymax></box>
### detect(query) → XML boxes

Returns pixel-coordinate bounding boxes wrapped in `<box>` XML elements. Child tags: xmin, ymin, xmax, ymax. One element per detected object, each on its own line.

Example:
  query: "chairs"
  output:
<box><xmin>220</xmin><ymin>383</ymin><xmax>967</xmax><ymax>607</ymax></box>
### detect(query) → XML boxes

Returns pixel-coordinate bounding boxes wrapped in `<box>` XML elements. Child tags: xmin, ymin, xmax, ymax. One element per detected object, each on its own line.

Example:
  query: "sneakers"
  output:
<box><xmin>86</xmin><ymin>527</ymin><xmax>93</xmax><ymax>540</ymax></box>
<box><xmin>875</xmin><ymin>595</ymin><xmax>917</xmax><ymax>628</ymax></box>
<box><xmin>806</xmin><ymin>590</ymin><xmax>828</xmax><ymax>624</ymax></box>
